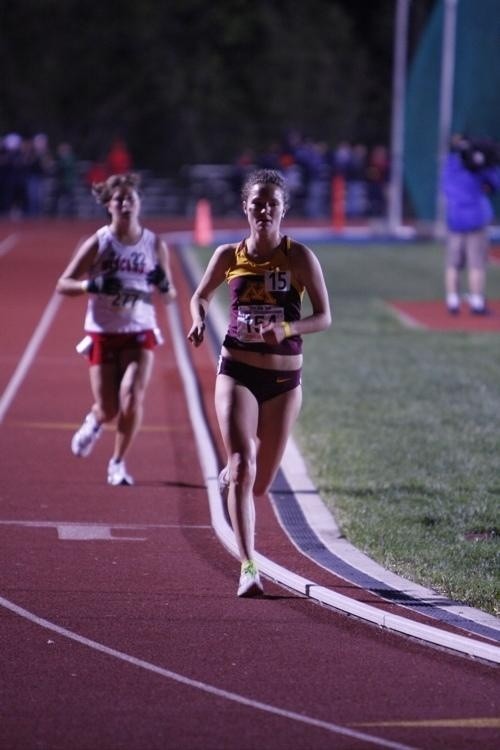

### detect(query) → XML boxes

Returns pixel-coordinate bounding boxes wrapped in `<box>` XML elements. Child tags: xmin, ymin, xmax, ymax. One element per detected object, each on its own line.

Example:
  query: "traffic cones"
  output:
<box><xmin>194</xmin><ymin>197</ymin><xmax>213</xmax><ymax>247</ymax></box>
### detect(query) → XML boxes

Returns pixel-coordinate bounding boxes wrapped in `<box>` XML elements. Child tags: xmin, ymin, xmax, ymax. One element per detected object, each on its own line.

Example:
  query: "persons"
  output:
<box><xmin>56</xmin><ymin>172</ymin><xmax>177</xmax><ymax>486</ymax></box>
<box><xmin>187</xmin><ymin>171</ymin><xmax>332</xmax><ymax>597</ymax></box>
<box><xmin>232</xmin><ymin>129</ymin><xmax>411</xmax><ymax>219</ymax></box>
<box><xmin>441</xmin><ymin>135</ymin><xmax>500</xmax><ymax>315</ymax></box>
<box><xmin>0</xmin><ymin>133</ymin><xmax>79</xmax><ymax>219</ymax></box>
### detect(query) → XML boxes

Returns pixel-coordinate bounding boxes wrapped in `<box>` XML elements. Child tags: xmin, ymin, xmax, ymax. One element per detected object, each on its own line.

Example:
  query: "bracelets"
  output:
<box><xmin>280</xmin><ymin>321</ymin><xmax>291</xmax><ymax>337</ymax></box>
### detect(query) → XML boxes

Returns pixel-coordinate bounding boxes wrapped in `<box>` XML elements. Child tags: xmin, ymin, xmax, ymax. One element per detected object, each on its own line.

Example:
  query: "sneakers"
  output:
<box><xmin>218</xmin><ymin>464</ymin><xmax>231</xmax><ymax>495</ymax></box>
<box><xmin>107</xmin><ymin>460</ymin><xmax>132</xmax><ymax>486</ymax></box>
<box><xmin>237</xmin><ymin>559</ymin><xmax>263</xmax><ymax>597</ymax></box>
<box><xmin>71</xmin><ymin>413</ymin><xmax>100</xmax><ymax>456</ymax></box>
